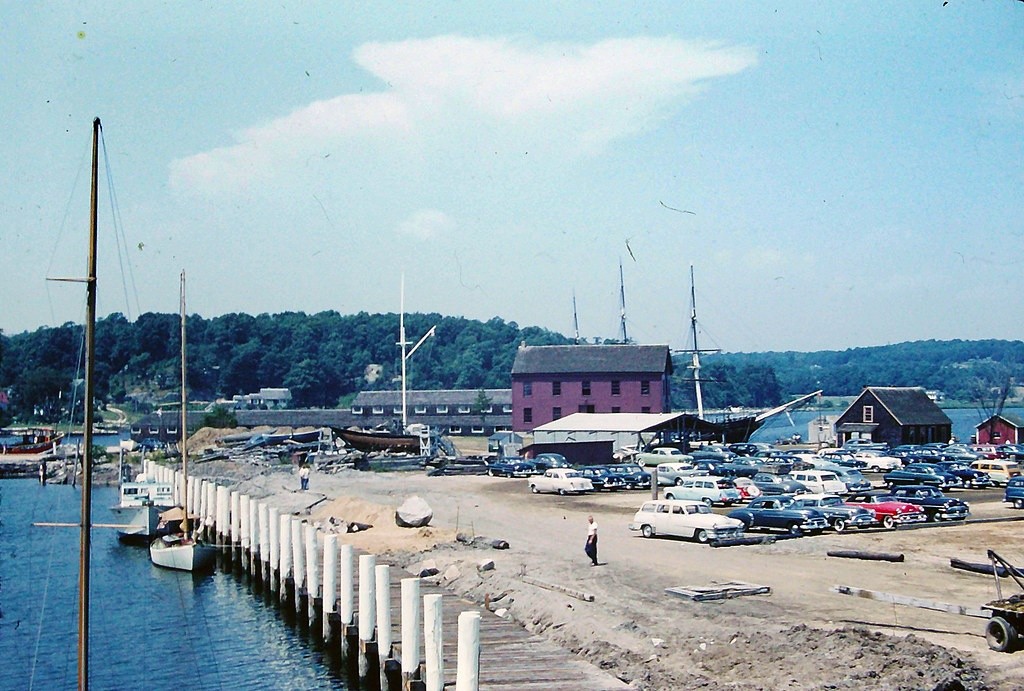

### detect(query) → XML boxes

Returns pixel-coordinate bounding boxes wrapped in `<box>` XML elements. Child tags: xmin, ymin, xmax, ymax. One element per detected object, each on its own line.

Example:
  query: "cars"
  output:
<box><xmin>635</xmin><ymin>442</ymin><xmax>872</xmax><ymax>507</ymax></box>
<box><xmin>528</xmin><ymin>453</ymin><xmax>572</xmax><ymax>475</ymax></box>
<box><xmin>488</xmin><ymin>456</ymin><xmax>536</xmax><ymax>478</ymax></box>
<box><xmin>602</xmin><ymin>464</ymin><xmax>661</xmax><ymax>490</ymax></box>
<box><xmin>891</xmin><ymin>485</ymin><xmax>971</xmax><ymax>522</ymax></box>
<box><xmin>1002</xmin><ymin>476</ymin><xmax>1024</xmax><ymax>509</ymax></box>
<box><xmin>840</xmin><ymin>438</ymin><xmax>1024</xmax><ymax>492</ymax></box>
<box><xmin>727</xmin><ymin>495</ymin><xmax>831</xmax><ymax>537</ymax></box>
<box><xmin>844</xmin><ymin>491</ymin><xmax>927</xmax><ymax>529</ymax></box>
<box><xmin>135</xmin><ymin>438</ymin><xmax>167</xmax><ymax>453</ymax></box>
<box><xmin>793</xmin><ymin>494</ymin><xmax>878</xmax><ymax>532</ymax></box>
<box><xmin>527</xmin><ymin>468</ymin><xmax>594</xmax><ymax>495</ymax></box>
<box><xmin>567</xmin><ymin>466</ymin><xmax>626</xmax><ymax>492</ymax></box>
<box><xmin>629</xmin><ymin>500</ymin><xmax>746</xmax><ymax>544</ymax></box>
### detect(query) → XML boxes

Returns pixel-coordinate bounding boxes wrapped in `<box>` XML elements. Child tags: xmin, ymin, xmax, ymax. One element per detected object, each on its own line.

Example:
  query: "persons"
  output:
<box><xmin>698</xmin><ymin>443</ymin><xmax>705</xmax><ymax>450</ymax></box>
<box><xmin>390</xmin><ymin>419</ymin><xmax>405</xmax><ymax>435</ymax></box>
<box><xmin>584</xmin><ymin>515</ymin><xmax>599</xmax><ymax>566</ymax></box>
<box><xmin>708</xmin><ymin>441</ymin><xmax>712</xmax><ymax>445</ymax></box>
<box><xmin>300</xmin><ymin>465</ymin><xmax>310</xmax><ymax>490</ymax></box>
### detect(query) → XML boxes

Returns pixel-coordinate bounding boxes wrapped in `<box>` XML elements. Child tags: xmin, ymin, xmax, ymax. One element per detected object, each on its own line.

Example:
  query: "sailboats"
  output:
<box><xmin>151</xmin><ymin>269</ymin><xmax>212</xmax><ymax>571</ymax></box>
<box><xmin>674</xmin><ymin>265</ymin><xmax>824</xmax><ymax>443</ymax></box>
<box><xmin>329</xmin><ymin>271</ymin><xmax>436</xmax><ymax>454</ymax></box>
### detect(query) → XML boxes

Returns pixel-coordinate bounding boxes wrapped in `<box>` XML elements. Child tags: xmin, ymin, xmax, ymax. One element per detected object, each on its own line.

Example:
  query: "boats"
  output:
<box><xmin>262</xmin><ymin>430</ymin><xmax>324</xmax><ymax>446</ymax></box>
<box><xmin>107</xmin><ymin>482</ymin><xmax>182</xmax><ymax>537</ymax></box>
<box><xmin>0</xmin><ymin>427</ymin><xmax>64</xmax><ymax>455</ymax></box>
